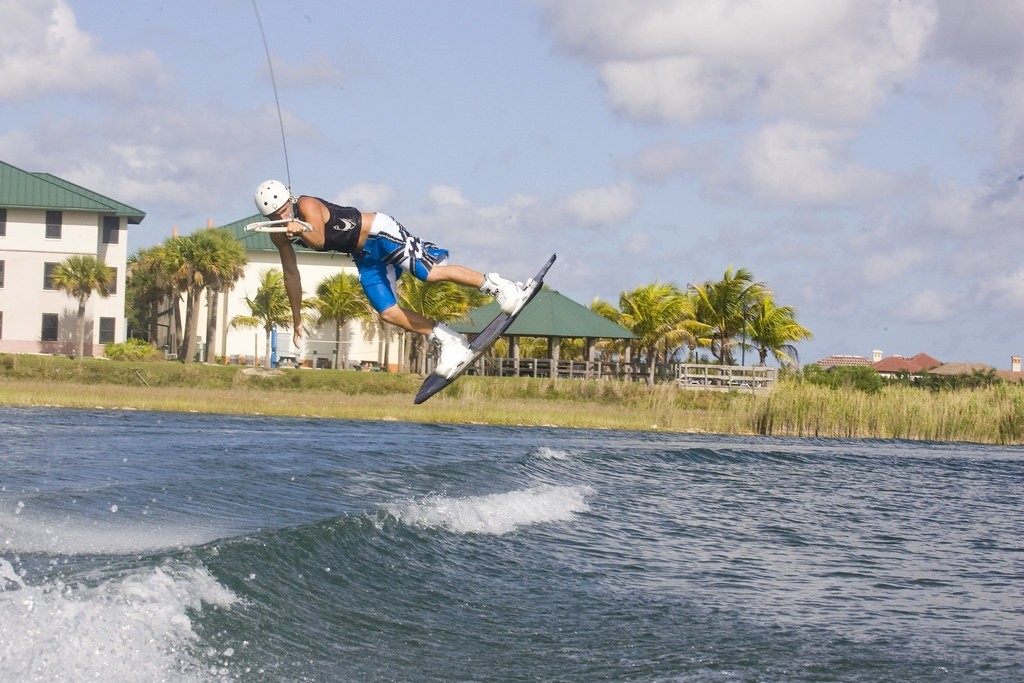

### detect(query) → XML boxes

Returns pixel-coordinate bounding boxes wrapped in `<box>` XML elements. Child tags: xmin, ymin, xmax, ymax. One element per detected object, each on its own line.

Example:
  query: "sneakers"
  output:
<box><xmin>479</xmin><ymin>272</ymin><xmax>524</xmax><ymax>314</ymax></box>
<box><xmin>427</xmin><ymin>322</ymin><xmax>472</xmax><ymax>378</ymax></box>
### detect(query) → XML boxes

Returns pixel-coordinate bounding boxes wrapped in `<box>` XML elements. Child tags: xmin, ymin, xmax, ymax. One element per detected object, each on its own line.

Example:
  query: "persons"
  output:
<box><xmin>253</xmin><ymin>179</ymin><xmax>524</xmax><ymax>379</ymax></box>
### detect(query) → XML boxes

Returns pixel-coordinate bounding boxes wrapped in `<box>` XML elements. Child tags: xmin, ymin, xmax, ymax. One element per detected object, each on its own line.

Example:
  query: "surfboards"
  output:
<box><xmin>415</xmin><ymin>252</ymin><xmax>556</xmax><ymax>403</ymax></box>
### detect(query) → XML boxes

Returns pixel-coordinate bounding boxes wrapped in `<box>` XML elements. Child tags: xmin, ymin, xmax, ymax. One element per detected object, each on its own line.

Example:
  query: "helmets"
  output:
<box><xmin>254</xmin><ymin>179</ymin><xmax>292</xmax><ymax>217</ymax></box>
